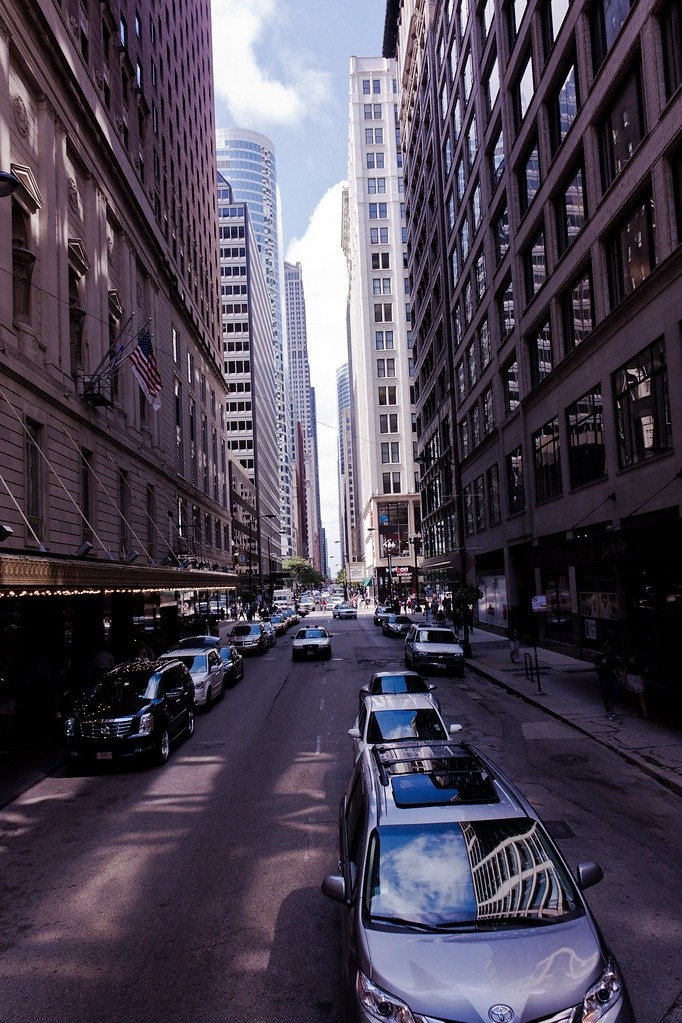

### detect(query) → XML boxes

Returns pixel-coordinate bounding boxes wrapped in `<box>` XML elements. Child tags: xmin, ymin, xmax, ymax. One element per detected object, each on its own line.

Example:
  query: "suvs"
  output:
<box><xmin>322</xmin><ymin>737</ymin><xmax>635</xmax><ymax>1022</ymax></box>
<box><xmin>157</xmin><ymin>635</ymin><xmax>227</xmax><ymax>712</ymax></box>
<box><xmin>227</xmin><ymin>621</ymin><xmax>269</xmax><ymax>656</ymax></box>
<box><xmin>404</xmin><ymin>623</ymin><xmax>465</xmax><ymax>678</ymax></box>
<box><xmin>62</xmin><ymin>658</ymin><xmax>197</xmax><ymax>773</ymax></box>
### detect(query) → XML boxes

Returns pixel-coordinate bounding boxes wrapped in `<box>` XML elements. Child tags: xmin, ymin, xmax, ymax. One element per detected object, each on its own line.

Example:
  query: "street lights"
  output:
<box><xmin>267</xmin><ymin>532</ymin><xmax>286</xmax><ymax>601</ymax></box>
<box><xmin>408</xmin><ymin>532</ymin><xmax>422</xmax><ymax>612</ymax></box>
<box><xmin>334</xmin><ymin>541</ymin><xmax>352</xmax><ymax>599</ymax></box>
<box><xmin>249</xmin><ymin>514</ymin><xmax>276</xmax><ymax>606</ymax></box>
<box><xmin>368</xmin><ymin>528</ymin><xmax>395</xmax><ymax>608</ymax></box>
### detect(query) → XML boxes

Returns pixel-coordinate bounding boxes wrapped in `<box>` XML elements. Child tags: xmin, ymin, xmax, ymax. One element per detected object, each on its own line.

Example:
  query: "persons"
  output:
<box><xmin>504</xmin><ymin>618</ymin><xmax>521</xmax><ymax>663</ymax></box>
<box><xmin>92</xmin><ymin>647</ymin><xmax>114</xmax><ymax>672</ymax></box>
<box><xmin>349</xmin><ymin>589</ymin><xmax>381</xmax><ymax>610</ymax></box>
<box><xmin>595</xmin><ymin>640</ymin><xmax>650</xmax><ymax>720</ymax></box>
<box><xmin>27</xmin><ymin>649</ymin><xmax>52</xmax><ymax>694</ymax></box>
<box><xmin>321</xmin><ymin>600</ymin><xmax>326</xmax><ymax>609</ymax></box>
<box><xmin>220</xmin><ymin>600</ymin><xmax>270</xmax><ymax>622</ymax></box>
<box><xmin>383</xmin><ymin>592</ymin><xmax>473</xmax><ymax>635</ymax></box>
<box><xmin>136</xmin><ymin>640</ymin><xmax>154</xmax><ymax>660</ymax></box>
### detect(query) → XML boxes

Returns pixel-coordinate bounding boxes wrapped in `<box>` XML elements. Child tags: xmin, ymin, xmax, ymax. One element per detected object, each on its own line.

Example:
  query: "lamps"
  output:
<box><xmin>0</xmin><ymin>525</ymin><xmax>14</xmax><ymax>542</ymax></box>
<box><xmin>127</xmin><ymin>550</ymin><xmax>140</xmax><ymax>563</ymax></box>
<box><xmin>182</xmin><ymin>559</ymin><xmax>192</xmax><ymax>567</ymax></box>
<box><xmin>159</xmin><ymin>556</ymin><xmax>172</xmax><ymax>566</ymax></box>
<box><xmin>76</xmin><ymin>541</ymin><xmax>93</xmax><ymax>556</ymax></box>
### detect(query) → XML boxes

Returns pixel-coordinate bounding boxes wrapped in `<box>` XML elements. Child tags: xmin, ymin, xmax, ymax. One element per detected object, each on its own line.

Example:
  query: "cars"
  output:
<box><xmin>264</xmin><ymin>609</ymin><xmax>301</xmax><ymax>637</ymax></box>
<box><xmin>264</xmin><ymin>621</ymin><xmax>277</xmax><ymax>648</ymax></box>
<box><xmin>347</xmin><ymin>693</ymin><xmax>464</xmax><ymax>766</ymax></box>
<box><xmin>382</xmin><ymin>615</ymin><xmax>414</xmax><ymax>638</ymax></box>
<box><xmin>359</xmin><ymin>669</ymin><xmax>442</xmax><ymax>714</ymax></box>
<box><xmin>290</xmin><ymin>625</ymin><xmax>333</xmax><ymax>661</ymax></box>
<box><xmin>219</xmin><ymin>646</ymin><xmax>245</xmax><ymax>689</ymax></box>
<box><xmin>374</xmin><ymin>607</ymin><xmax>397</xmax><ymax>626</ymax></box>
<box><xmin>298</xmin><ymin>588</ymin><xmax>357</xmax><ymax>619</ymax></box>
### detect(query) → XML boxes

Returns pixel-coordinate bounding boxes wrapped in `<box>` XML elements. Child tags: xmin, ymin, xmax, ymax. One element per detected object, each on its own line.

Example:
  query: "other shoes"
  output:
<box><xmin>608</xmin><ymin>711</ymin><xmax>615</xmax><ymax>720</ymax></box>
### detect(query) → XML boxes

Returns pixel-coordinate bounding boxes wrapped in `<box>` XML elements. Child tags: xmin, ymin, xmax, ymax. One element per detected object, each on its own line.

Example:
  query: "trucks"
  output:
<box><xmin>272</xmin><ymin>589</ymin><xmax>294</xmax><ymax>606</ymax></box>
